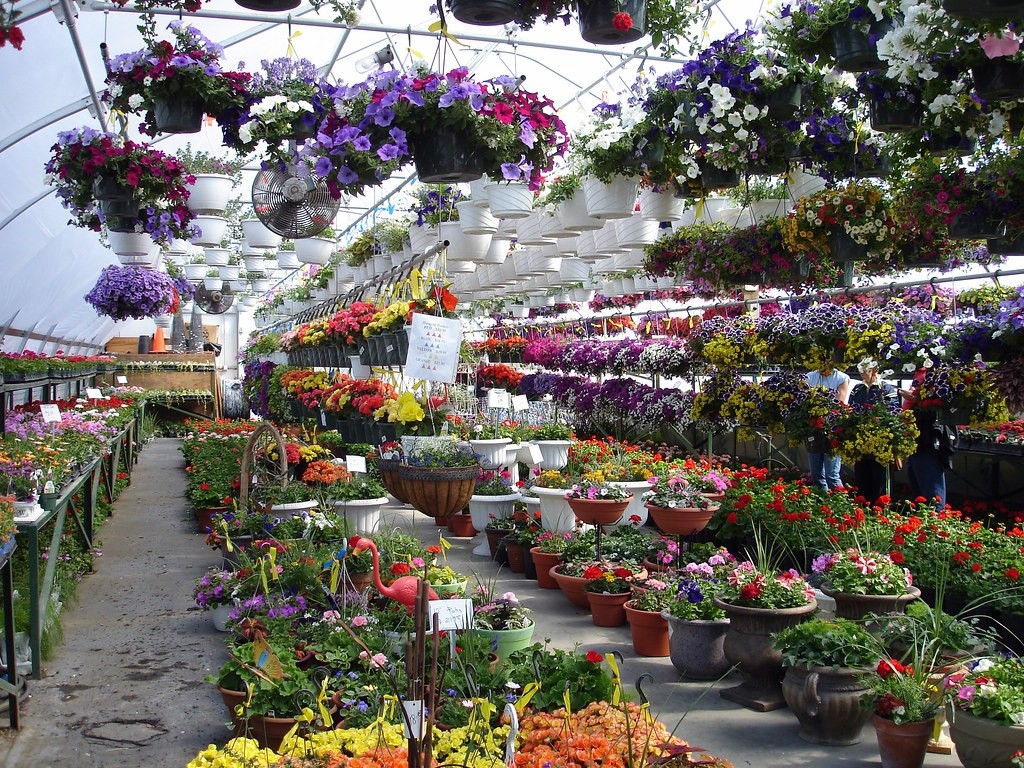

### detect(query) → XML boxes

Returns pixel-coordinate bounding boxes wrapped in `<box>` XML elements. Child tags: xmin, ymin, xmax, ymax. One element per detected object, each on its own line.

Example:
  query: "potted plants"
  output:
<box><xmin>0</xmin><ymin>586</ymin><xmax>65</xmax><ymax>665</ymax></box>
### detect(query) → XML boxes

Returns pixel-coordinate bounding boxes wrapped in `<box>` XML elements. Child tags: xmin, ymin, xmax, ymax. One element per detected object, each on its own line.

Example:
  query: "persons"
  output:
<box><xmin>898</xmin><ymin>367</ymin><xmax>946</xmax><ymax>513</ymax></box>
<box><xmin>848</xmin><ymin>357</ymin><xmax>899</xmax><ymax>507</ymax></box>
<box><xmin>802</xmin><ymin>353</ymin><xmax>851</xmax><ymax>491</ymax></box>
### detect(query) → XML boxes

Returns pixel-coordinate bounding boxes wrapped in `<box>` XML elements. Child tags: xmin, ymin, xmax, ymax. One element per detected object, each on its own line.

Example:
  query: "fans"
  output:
<box><xmin>251</xmin><ymin>158</ymin><xmax>341</xmax><ymax>238</ymax></box>
<box><xmin>194</xmin><ymin>277</ymin><xmax>234</xmax><ymax>315</ymax></box>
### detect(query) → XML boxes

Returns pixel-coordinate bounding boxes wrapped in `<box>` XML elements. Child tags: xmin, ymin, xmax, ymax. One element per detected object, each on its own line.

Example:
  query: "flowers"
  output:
<box><xmin>180</xmin><ymin>375</ymin><xmax>1024</xmax><ymax>768</ymax></box>
<box><xmin>0</xmin><ymin>351</ymin><xmax>144</xmax><ymax>591</ymax></box>
<box><xmin>0</xmin><ymin>0</ymin><xmax>1024</xmax><ymax>338</ymax></box>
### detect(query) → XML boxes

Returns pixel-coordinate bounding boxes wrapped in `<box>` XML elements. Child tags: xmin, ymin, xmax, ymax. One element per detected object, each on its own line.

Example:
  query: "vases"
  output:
<box><xmin>486</xmin><ymin>181</ymin><xmax>535</xmax><ymax>219</ymax></box>
<box><xmin>12</xmin><ymin>499</ymin><xmax>36</xmax><ymax>518</ymax></box>
<box><xmin>944</xmin><ymin>702</ymin><xmax>1024</xmax><ymax>768</ymax></box>
<box><xmin>242</xmin><ymin>331</ymin><xmax>718</xmax><ymax>627</ymax></box>
<box><xmin>874</xmin><ymin>710</ymin><xmax>935</xmax><ymax>768</ymax></box>
<box><xmin>820</xmin><ymin>583</ymin><xmax>920</xmax><ymax>642</ymax></box>
<box><xmin>51</xmin><ymin>366</ymin><xmax>94</xmax><ymax>377</ymax></box>
<box><xmin>623</xmin><ymin>602</ymin><xmax>671</xmax><ymax>656</ymax></box>
<box><xmin>585</xmin><ymin>169</ymin><xmax>641</xmax><ymax>217</ymax></box>
<box><xmin>711</xmin><ymin>594</ymin><xmax>816</xmax><ymax>712</ymax></box>
<box><xmin>5</xmin><ymin>370</ymin><xmax>49</xmax><ymax>381</ymax></box>
<box><xmin>155</xmin><ymin>87</ymin><xmax>205</xmax><ymax>132</ymax></box>
<box><xmin>183</xmin><ymin>174</ymin><xmax>236</xmax><ymax>213</ymax></box>
<box><xmin>412</xmin><ymin>122</ymin><xmax>482</xmax><ymax>183</ymax></box>
<box><xmin>643</xmin><ymin>181</ymin><xmax>685</xmax><ymax>222</ymax></box>
<box><xmin>664</xmin><ymin>608</ymin><xmax>730</xmax><ymax>682</ymax></box>
<box><xmin>783</xmin><ymin>659</ymin><xmax>880</xmax><ymax>747</ymax></box>
